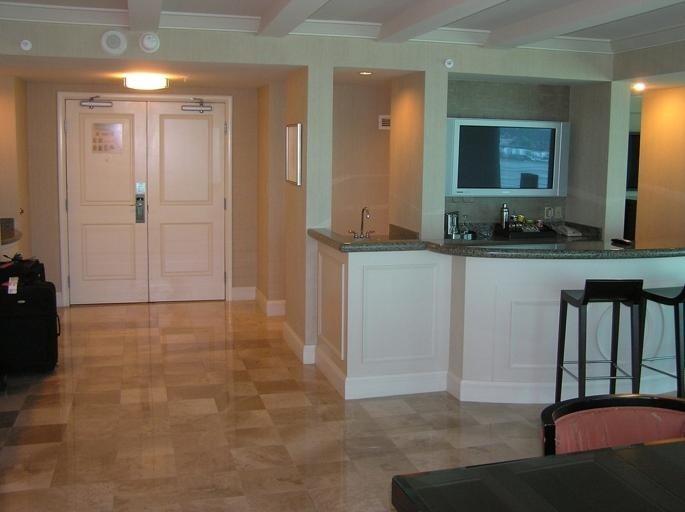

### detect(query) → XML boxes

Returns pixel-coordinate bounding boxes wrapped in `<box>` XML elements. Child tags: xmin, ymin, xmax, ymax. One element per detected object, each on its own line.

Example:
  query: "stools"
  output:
<box><xmin>643</xmin><ymin>283</ymin><xmax>685</xmax><ymax>398</ymax></box>
<box><xmin>556</xmin><ymin>278</ymin><xmax>644</xmax><ymax>402</ymax></box>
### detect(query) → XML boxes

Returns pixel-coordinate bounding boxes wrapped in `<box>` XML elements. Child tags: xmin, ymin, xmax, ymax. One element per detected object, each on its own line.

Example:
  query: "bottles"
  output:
<box><xmin>502</xmin><ymin>203</ymin><xmax>509</xmax><ymax>231</ymax></box>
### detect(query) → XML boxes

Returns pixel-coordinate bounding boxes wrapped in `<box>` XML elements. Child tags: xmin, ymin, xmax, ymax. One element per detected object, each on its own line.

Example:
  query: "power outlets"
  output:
<box><xmin>555</xmin><ymin>206</ymin><xmax>561</xmax><ymax>218</ymax></box>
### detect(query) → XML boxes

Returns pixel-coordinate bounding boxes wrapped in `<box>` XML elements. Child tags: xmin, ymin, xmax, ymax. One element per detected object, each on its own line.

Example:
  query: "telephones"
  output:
<box><xmin>551</xmin><ymin>225</ymin><xmax>583</xmax><ymax>237</ymax></box>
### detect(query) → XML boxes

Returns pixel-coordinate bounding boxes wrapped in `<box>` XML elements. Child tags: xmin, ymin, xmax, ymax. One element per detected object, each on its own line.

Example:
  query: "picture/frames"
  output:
<box><xmin>284</xmin><ymin>123</ymin><xmax>302</xmax><ymax>186</ymax></box>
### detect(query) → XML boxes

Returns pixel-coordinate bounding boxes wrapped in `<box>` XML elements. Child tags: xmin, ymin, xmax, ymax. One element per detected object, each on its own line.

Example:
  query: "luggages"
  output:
<box><xmin>1</xmin><ymin>254</ymin><xmax>62</xmax><ymax>375</ymax></box>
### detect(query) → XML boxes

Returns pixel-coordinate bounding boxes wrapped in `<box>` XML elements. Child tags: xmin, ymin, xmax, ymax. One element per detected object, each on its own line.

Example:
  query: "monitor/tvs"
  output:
<box><xmin>443</xmin><ymin>117</ymin><xmax>571</xmax><ymax>198</ymax></box>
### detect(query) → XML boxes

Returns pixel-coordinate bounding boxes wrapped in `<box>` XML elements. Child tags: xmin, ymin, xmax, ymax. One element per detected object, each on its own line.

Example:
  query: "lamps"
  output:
<box><xmin>124</xmin><ymin>73</ymin><xmax>170</xmax><ymax>91</ymax></box>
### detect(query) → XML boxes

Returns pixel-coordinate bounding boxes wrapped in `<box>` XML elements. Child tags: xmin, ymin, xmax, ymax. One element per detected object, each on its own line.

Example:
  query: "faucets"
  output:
<box><xmin>361</xmin><ymin>206</ymin><xmax>370</xmax><ymax>234</ymax></box>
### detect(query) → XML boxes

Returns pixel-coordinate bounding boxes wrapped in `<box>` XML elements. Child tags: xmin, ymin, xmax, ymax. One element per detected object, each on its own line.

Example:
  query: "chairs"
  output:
<box><xmin>540</xmin><ymin>394</ymin><xmax>685</xmax><ymax>457</ymax></box>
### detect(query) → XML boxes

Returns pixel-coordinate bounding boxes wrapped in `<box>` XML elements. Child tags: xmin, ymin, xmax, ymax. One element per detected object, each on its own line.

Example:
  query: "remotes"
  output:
<box><xmin>612</xmin><ymin>238</ymin><xmax>632</xmax><ymax>244</ymax></box>
